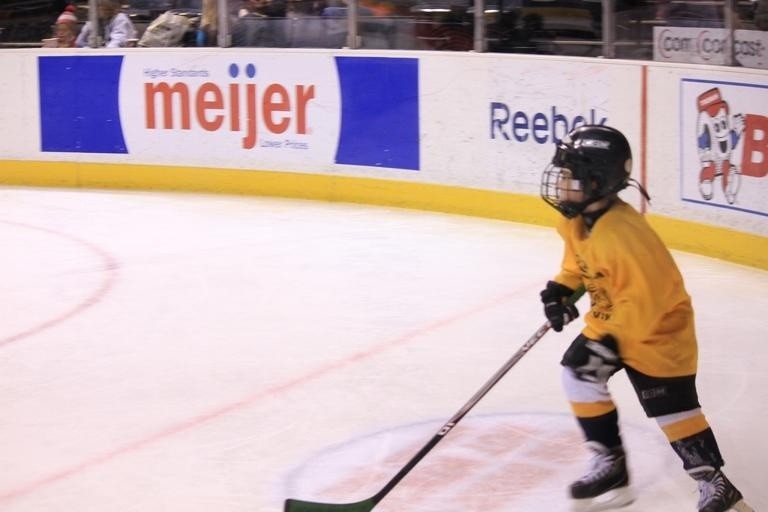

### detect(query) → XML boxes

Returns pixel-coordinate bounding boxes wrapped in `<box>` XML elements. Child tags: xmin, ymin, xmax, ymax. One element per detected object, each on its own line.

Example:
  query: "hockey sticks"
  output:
<box><xmin>284</xmin><ymin>282</ymin><xmax>590</xmax><ymax>512</ymax></box>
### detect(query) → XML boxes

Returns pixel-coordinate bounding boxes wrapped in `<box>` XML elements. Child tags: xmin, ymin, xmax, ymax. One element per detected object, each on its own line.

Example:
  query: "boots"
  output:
<box><xmin>685</xmin><ymin>460</ymin><xmax>744</xmax><ymax>510</ymax></box>
<box><xmin>567</xmin><ymin>436</ymin><xmax>630</xmax><ymax>501</ymax></box>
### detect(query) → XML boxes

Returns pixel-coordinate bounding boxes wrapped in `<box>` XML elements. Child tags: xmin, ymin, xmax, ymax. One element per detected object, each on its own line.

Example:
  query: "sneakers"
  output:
<box><xmin>699</xmin><ymin>159</ymin><xmax>718</xmax><ymax>183</ymax></box>
<box><xmin>721</xmin><ymin>160</ymin><xmax>735</xmax><ymax>192</ymax></box>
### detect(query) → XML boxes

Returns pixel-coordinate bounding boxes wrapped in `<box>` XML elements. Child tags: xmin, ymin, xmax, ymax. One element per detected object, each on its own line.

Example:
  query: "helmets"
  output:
<box><xmin>554</xmin><ymin>121</ymin><xmax>632</xmax><ymax>199</ymax></box>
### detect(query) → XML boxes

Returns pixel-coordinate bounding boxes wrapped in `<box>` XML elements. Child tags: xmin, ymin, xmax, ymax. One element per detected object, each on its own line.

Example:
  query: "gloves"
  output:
<box><xmin>540</xmin><ymin>280</ymin><xmax>582</xmax><ymax>333</ymax></box>
<box><xmin>567</xmin><ymin>333</ymin><xmax>626</xmax><ymax>384</ymax></box>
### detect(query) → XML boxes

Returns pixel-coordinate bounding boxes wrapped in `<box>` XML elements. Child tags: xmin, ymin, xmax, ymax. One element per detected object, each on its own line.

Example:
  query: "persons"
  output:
<box><xmin>74</xmin><ymin>1</ymin><xmax>139</xmax><ymax>47</ymax></box>
<box><xmin>539</xmin><ymin>124</ymin><xmax>746</xmax><ymax>512</ymax></box>
<box><xmin>52</xmin><ymin>5</ymin><xmax>77</xmax><ymax>47</ymax></box>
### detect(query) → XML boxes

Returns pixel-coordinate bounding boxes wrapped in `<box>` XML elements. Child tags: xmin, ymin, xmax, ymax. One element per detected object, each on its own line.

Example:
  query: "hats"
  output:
<box><xmin>52</xmin><ymin>12</ymin><xmax>79</xmax><ymax>28</ymax></box>
<box><xmin>696</xmin><ymin>88</ymin><xmax>730</xmax><ymax>118</ymax></box>
